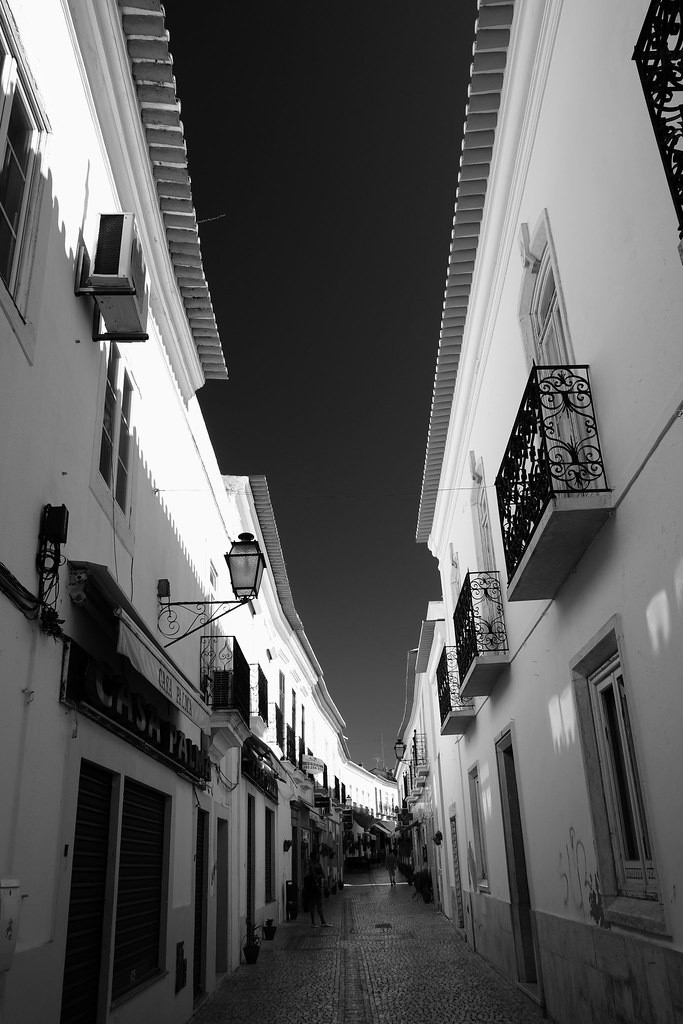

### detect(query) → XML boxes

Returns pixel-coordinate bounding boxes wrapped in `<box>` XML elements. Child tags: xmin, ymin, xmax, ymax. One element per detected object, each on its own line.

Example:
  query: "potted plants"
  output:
<box><xmin>288</xmin><ymin>900</ymin><xmax>301</xmax><ymax>921</ymax></box>
<box><xmin>323</xmin><ymin>886</ymin><xmax>331</xmax><ymax>898</ymax></box>
<box><xmin>240</xmin><ymin>919</ymin><xmax>263</xmax><ymax>964</ymax></box>
<box><xmin>338</xmin><ymin>881</ymin><xmax>345</xmax><ymax>891</ymax></box>
<box><xmin>411</xmin><ymin>869</ymin><xmax>433</xmax><ymax>905</ymax></box>
<box><xmin>329</xmin><ymin>885</ymin><xmax>338</xmax><ymax>895</ymax></box>
<box><xmin>262</xmin><ymin>918</ymin><xmax>278</xmax><ymax>941</ymax></box>
<box><xmin>396</xmin><ymin>860</ymin><xmax>414</xmax><ymax>887</ymax></box>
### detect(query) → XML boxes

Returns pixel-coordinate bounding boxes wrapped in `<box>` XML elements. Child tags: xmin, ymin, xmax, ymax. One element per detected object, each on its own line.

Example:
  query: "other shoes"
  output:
<box><xmin>321</xmin><ymin>923</ymin><xmax>333</xmax><ymax>927</ymax></box>
<box><xmin>311</xmin><ymin>924</ymin><xmax>319</xmax><ymax>927</ymax></box>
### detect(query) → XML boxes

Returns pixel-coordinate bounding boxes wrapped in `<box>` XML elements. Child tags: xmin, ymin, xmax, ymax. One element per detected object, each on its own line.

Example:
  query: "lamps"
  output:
<box><xmin>393</xmin><ymin>738</ymin><xmax>425</xmax><ymax>772</ymax></box>
<box><xmin>156</xmin><ymin>532</ymin><xmax>267</xmax><ymax>649</ymax></box>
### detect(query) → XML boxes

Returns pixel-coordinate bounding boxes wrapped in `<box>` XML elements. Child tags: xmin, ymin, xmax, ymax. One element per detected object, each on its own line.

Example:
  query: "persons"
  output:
<box><xmin>303</xmin><ymin>849</ymin><xmax>334</xmax><ymax>929</ymax></box>
<box><xmin>385</xmin><ymin>849</ymin><xmax>398</xmax><ymax>887</ymax></box>
<box><xmin>376</xmin><ymin>849</ymin><xmax>382</xmax><ymax>866</ymax></box>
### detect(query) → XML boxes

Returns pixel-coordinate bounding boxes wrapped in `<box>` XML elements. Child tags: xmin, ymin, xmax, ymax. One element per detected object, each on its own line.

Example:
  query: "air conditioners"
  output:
<box><xmin>210</xmin><ymin>667</ymin><xmax>235</xmax><ymax>711</ymax></box>
<box><xmin>87</xmin><ymin>211</ymin><xmax>152</xmax><ymax>341</ymax></box>
